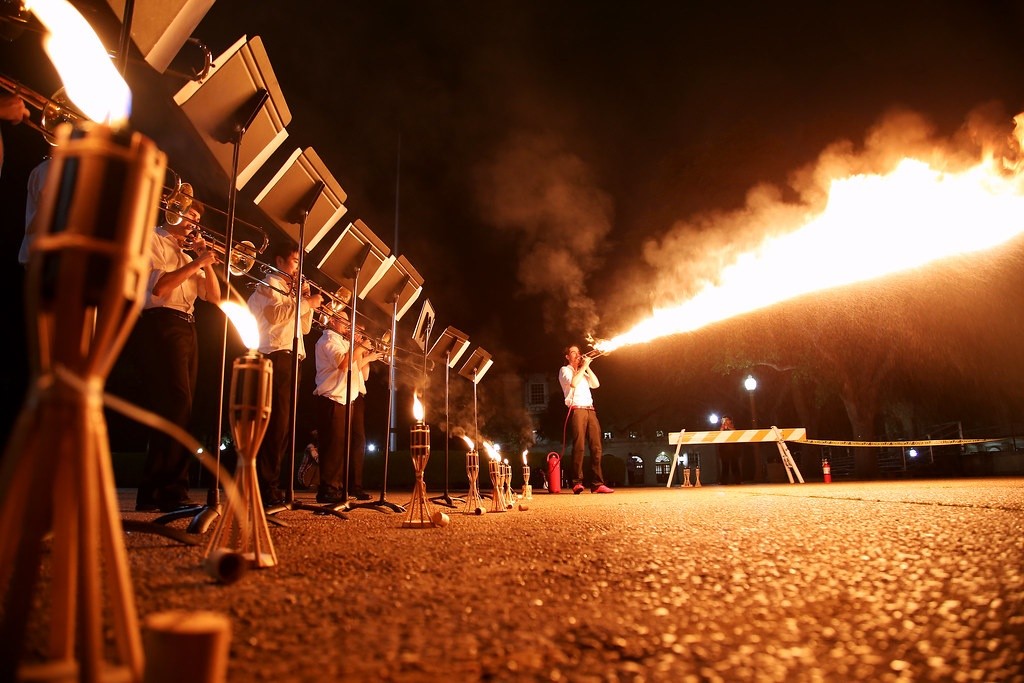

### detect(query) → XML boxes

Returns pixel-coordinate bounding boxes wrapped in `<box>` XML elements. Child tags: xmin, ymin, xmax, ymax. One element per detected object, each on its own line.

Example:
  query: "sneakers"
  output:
<box><xmin>573</xmin><ymin>483</ymin><xmax>583</xmax><ymax>494</ymax></box>
<box><xmin>592</xmin><ymin>485</ymin><xmax>614</xmax><ymax>494</ymax></box>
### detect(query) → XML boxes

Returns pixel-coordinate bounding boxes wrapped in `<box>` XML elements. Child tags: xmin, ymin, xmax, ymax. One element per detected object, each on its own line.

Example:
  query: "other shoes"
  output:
<box><xmin>349</xmin><ymin>494</ymin><xmax>373</xmax><ymax>500</ymax></box>
<box><xmin>262</xmin><ymin>494</ymin><xmax>291</xmax><ymax>506</ymax></box>
<box><xmin>135</xmin><ymin>500</ymin><xmax>203</xmax><ymax>513</ymax></box>
<box><xmin>316</xmin><ymin>495</ymin><xmax>344</xmax><ymax>503</ymax></box>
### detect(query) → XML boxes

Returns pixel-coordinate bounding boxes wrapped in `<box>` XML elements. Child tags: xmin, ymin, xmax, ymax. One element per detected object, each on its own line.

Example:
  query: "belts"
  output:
<box><xmin>572</xmin><ymin>406</ymin><xmax>594</xmax><ymax>411</ymax></box>
<box><xmin>275</xmin><ymin>350</ymin><xmax>302</xmax><ymax>362</ymax></box>
<box><xmin>359</xmin><ymin>393</ymin><xmax>364</xmax><ymax>398</ymax></box>
<box><xmin>142</xmin><ymin>307</ymin><xmax>195</xmax><ymax>323</ymax></box>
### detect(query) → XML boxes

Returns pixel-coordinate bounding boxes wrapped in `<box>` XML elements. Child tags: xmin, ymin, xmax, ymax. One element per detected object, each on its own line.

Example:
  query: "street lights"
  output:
<box><xmin>744</xmin><ymin>375</ymin><xmax>764</xmax><ymax>484</ymax></box>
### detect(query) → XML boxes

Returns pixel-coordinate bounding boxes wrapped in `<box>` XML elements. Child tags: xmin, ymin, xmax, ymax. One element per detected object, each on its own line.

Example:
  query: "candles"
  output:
<box><xmin>462</xmin><ymin>435</ymin><xmax>479</xmax><ymax>472</ymax></box>
<box><xmin>410</xmin><ymin>392</ymin><xmax>430</xmax><ymax>456</ymax></box>
<box><xmin>218</xmin><ymin>303</ymin><xmax>272</xmax><ymax>429</ymax></box>
<box><xmin>522</xmin><ymin>450</ymin><xmax>530</xmax><ymax>479</ymax></box>
<box><xmin>483</xmin><ymin>442</ymin><xmax>512</xmax><ymax>481</ymax></box>
<box><xmin>24</xmin><ymin>0</ymin><xmax>160</xmax><ymax>313</ymax></box>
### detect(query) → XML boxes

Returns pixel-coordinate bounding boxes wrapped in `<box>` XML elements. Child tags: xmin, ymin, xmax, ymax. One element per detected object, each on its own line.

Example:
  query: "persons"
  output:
<box><xmin>558</xmin><ymin>345</ymin><xmax>616</xmax><ymax>493</ymax></box>
<box><xmin>246</xmin><ymin>238</ymin><xmax>306</xmax><ymax>502</ymax></box>
<box><xmin>314</xmin><ymin>311</ymin><xmax>384</xmax><ymax>504</ymax></box>
<box><xmin>296</xmin><ymin>431</ymin><xmax>320</xmax><ymax>493</ymax></box>
<box><xmin>716</xmin><ymin>414</ymin><xmax>743</xmax><ymax>485</ymax></box>
<box><xmin>134</xmin><ymin>198</ymin><xmax>221</xmax><ymax>516</ymax></box>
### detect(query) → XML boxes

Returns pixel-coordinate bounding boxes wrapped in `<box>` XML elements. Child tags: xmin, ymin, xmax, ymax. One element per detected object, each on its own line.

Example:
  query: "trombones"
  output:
<box><xmin>1</xmin><ymin>78</ymin><xmax>441</xmax><ymax>384</ymax></box>
